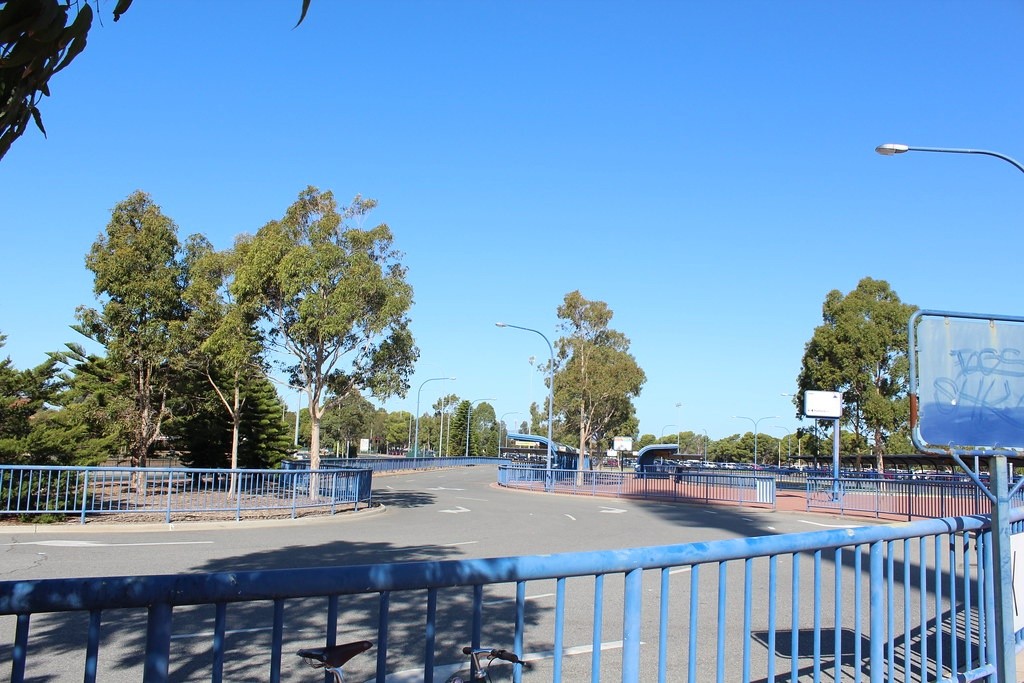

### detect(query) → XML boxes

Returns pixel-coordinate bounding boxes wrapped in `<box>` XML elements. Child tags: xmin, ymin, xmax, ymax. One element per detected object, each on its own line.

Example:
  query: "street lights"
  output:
<box><xmin>466</xmin><ymin>398</ymin><xmax>496</xmax><ymax>456</ymax></box>
<box><xmin>414</xmin><ymin>377</ymin><xmax>456</xmax><ymax>457</ymax></box>
<box><xmin>732</xmin><ymin>416</ymin><xmax>780</xmax><ymax>475</ymax></box>
<box><xmin>498</xmin><ymin>411</ymin><xmax>518</xmax><ymax>458</ymax></box>
<box><xmin>781</xmin><ymin>392</ymin><xmax>817</xmax><ymax>479</ymax></box>
<box><xmin>494</xmin><ymin>323</ymin><xmax>554</xmax><ymax>492</ymax></box>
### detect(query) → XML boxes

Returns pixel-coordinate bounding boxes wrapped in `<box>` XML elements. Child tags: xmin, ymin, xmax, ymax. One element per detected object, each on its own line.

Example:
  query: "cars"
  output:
<box><xmin>591</xmin><ymin>457</ymin><xmax>991</xmax><ymax>481</ymax></box>
